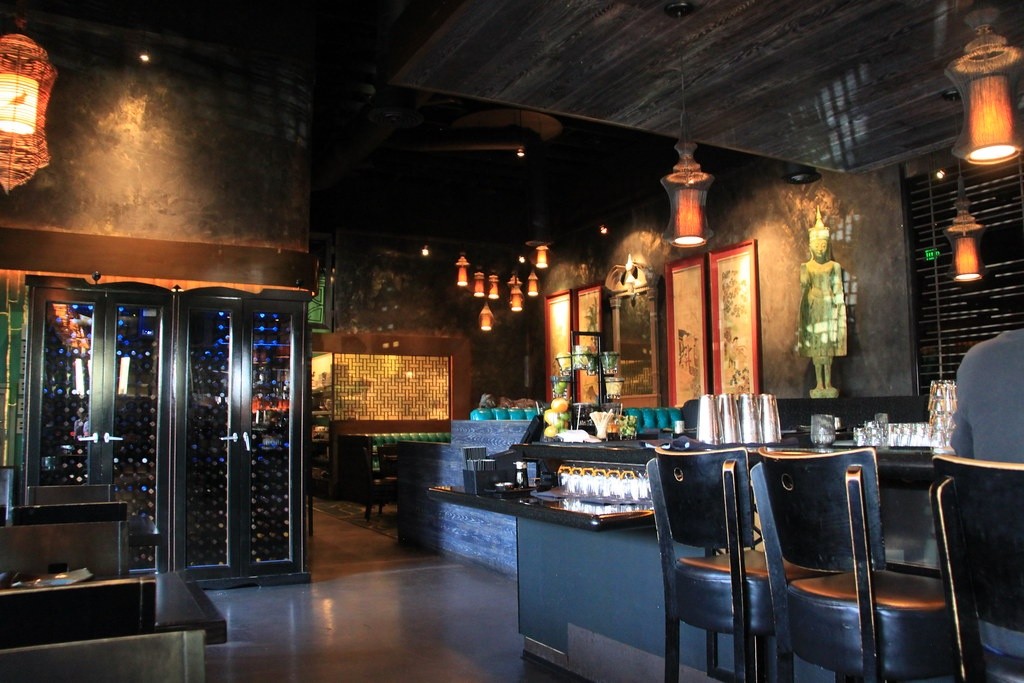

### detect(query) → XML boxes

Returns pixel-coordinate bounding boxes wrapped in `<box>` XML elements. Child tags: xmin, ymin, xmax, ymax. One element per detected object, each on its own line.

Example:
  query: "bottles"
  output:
<box><xmin>34</xmin><ymin>300</ymin><xmax>293</xmax><ymax>574</ymax></box>
<box><xmin>854</xmin><ymin>378</ymin><xmax>960</xmax><ymax>450</ymax></box>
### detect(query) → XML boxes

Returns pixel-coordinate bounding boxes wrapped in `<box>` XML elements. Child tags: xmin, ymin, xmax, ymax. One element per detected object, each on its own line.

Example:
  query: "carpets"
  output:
<box><xmin>306</xmin><ymin>493</ymin><xmax>398</xmax><ymax>539</ymax></box>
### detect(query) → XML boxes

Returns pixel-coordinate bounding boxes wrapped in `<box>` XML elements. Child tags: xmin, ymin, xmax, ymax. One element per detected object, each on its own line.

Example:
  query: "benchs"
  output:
<box><xmin>338</xmin><ymin>432</ymin><xmax>450</xmax><ymax>506</ymax></box>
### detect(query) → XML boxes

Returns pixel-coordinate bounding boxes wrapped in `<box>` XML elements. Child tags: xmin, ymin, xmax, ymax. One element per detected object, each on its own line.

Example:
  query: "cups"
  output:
<box><xmin>674</xmin><ymin>420</ymin><xmax>685</xmax><ymax>434</ymax></box>
<box><xmin>556</xmin><ymin>471</ymin><xmax>653</xmax><ymax>504</ymax></box>
<box><xmin>811</xmin><ymin>414</ymin><xmax>836</xmax><ymax>447</ymax></box>
<box><xmin>695</xmin><ymin>392</ymin><xmax>784</xmax><ymax>448</ymax></box>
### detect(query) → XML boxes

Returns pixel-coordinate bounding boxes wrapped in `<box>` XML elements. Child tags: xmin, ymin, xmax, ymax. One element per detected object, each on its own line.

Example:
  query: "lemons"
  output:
<box><xmin>554</xmin><ymin>381</ymin><xmax>567</xmax><ymax>394</ymax></box>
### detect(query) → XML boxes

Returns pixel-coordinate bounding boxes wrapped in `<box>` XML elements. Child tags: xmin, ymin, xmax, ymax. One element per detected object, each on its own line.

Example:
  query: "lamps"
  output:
<box><xmin>535</xmin><ymin>244</ymin><xmax>549</xmax><ymax>270</ymax></box>
<box><xmin>943</xmin><ymin>90</ymin><xmax>987</xmax><ymax>285</ymax></box>
<box><xmin>506</xmin><ymin>267</ymin><xmax>525</xmax><ymax>312</ymax></box>
<box><xmin>479</xmin><ymin>302</ymin><xmax>494</xmax><ymax>331</ymax></box>
<box><xmin>455</xmin><ymin>249</ymin><xmax>470</xmax><ymax>286</ymax></box>
<box><xmin>489</xmin><ymin>270</ymin><xmax>501</xmax><ymax>299</ymax></box>
<box><xmin>527</xmin><ymin>267</ymin><xmax>539</xmax><ymax>298</ymax></box>
<box><xmin>473</xmin><ymin>264</ymin><xmax>485</xmax><ymax>297</ymax></box>
<box><xmin>0</xmin><ymin>10</ymin><xmax>60</xmax><ymax>197</ymax></box>
<box><xmin>780</xmin><ymin>162</ymin><xmax>822</xmax><ymax>183</ymax></box>
<box><xmin>658</xmin><ymin>2</ymin><xmax>716</xmax><ymax>247</ymax></box>
<box><xmin>944</xmin><ymin>0</ymin><xmax>1024</xmax><ymax>165</ymax></box>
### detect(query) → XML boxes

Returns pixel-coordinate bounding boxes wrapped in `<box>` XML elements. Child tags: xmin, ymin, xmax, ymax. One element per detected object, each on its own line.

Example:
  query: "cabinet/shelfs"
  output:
<box><xmin>311</xmin><ymin>385</ymin><xmax>332</xmax><ymax>496</ymax></box>
<box><xmin>25</xmin><ymin>270</ymin><xmax>315</xmax><ymax>589</ymax></box>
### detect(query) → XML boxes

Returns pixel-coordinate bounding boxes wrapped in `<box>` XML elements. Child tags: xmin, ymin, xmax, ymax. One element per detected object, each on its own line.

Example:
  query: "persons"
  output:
<box><xmin>951</xmin><ymin>330</ymin><xmax>1024</xmax><ymax>663</ymax></box>
<box><xmin>799</xmin><ymin>205</ymin><xmax>848</xmax><ymax>399</ymax></box>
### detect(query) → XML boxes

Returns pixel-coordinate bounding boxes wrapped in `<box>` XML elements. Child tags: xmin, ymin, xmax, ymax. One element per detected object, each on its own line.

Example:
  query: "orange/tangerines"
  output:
<box><xmin>543</xmin><ymin>397</ymin><xmax>570</xmax><ymax>438</ymax></box>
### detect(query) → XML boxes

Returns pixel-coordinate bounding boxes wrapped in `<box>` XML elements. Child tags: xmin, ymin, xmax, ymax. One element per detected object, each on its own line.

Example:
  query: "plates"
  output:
<box><xmin>495</xmin><ymin>482</ymin><xmax>514</xmax><ymax>491</ymax></box>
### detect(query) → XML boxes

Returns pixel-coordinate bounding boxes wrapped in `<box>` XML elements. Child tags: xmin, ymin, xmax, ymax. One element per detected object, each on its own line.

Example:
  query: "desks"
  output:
<box><xmin>0</xmin><ymin>569</ymin><xmax>227</xmax><ymax>650</ymax></box>
<box><xmin>7</xmin><ymin>516</ymin><xmax>162</xmax><ymax>558</ymax></box>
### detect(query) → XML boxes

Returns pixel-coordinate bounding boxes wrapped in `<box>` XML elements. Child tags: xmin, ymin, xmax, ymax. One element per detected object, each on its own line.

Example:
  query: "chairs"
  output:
<box><xmin>365</xmin><ymin>435</ymin><xmax>398</xmax><ymax>524</ymax></box>
<box><xmin>1</xmin><ymin>483</ymin><xmax>207</xmax><ymax>683</ymax></box>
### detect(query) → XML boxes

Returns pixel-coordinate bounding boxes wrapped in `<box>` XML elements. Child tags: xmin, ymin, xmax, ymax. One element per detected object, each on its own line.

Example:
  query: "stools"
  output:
<box><xmin>644</xmin><ymin>443</ymin><xmax>1024</xmax><ymax>683</ymax></box>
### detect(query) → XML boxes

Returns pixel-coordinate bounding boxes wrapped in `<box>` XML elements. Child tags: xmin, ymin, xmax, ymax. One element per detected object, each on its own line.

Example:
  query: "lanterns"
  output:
<box><xmin>0</xmin><ymin>30</ymin><xmax>56</xmax><ymax>196</ymax></box>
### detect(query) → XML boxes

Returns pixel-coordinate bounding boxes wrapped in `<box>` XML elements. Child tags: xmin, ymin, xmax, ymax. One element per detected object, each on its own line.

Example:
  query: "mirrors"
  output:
<box><xmin>602</xmin><ymin>252</ymin><xmax>661</xmax><ymax>411</ymax></box>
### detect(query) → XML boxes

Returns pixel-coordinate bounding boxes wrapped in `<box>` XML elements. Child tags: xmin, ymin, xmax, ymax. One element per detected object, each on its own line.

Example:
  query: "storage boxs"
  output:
<box><xmin>462</xmin><ymin>469</ymin><xmax>507</xmax><ymax>496</ymax></box>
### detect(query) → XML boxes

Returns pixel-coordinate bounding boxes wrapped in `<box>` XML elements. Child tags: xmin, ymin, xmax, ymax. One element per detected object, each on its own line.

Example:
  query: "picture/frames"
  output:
<box><xmin>663</xmin><ymin>251</ymin><xmax>712</xmax><ymax>408</ymax></box>
<box><xmin>707</xmin><ymin>239</ymin><xmax>762</xmax><ymax>396</ymax></box>
<box><xmin>542</xmin><ymin>279</ymin><xmax>602</xmax><ymax>406</ymax></box>
